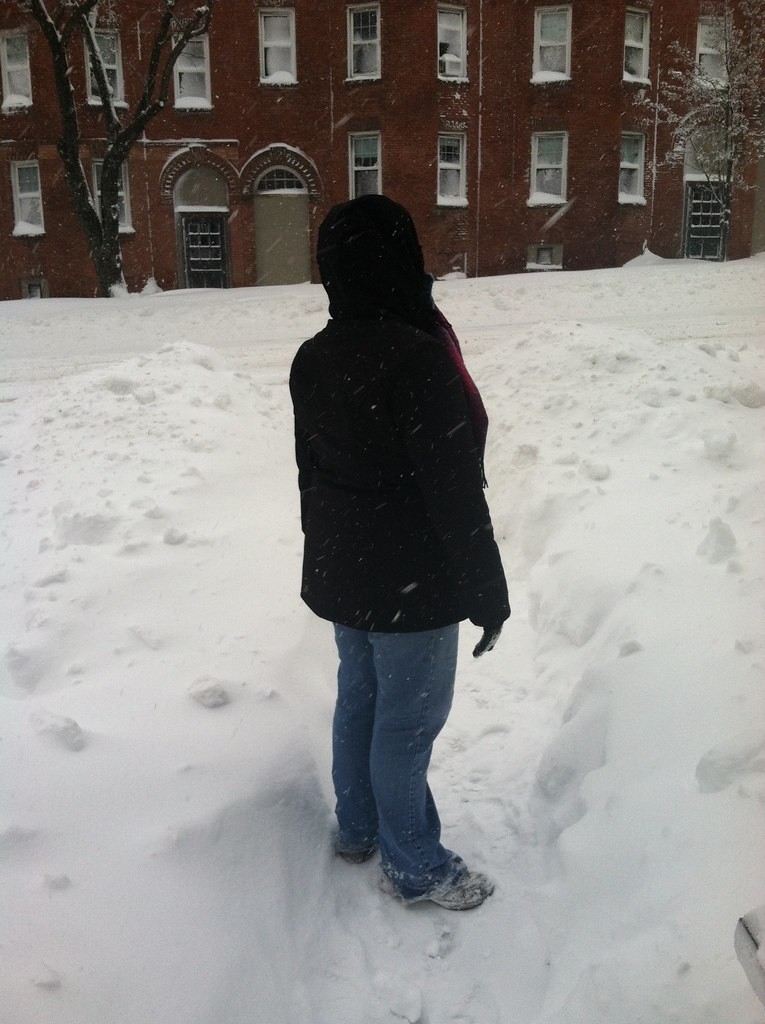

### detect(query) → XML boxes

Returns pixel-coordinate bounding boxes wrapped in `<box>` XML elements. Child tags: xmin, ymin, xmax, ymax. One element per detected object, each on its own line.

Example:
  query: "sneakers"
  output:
<box><xmin>378</xmin><ymin>871</ymin><xmax>495</xmax><ymax>910</ymax></box>
<box><xmin>339</xmin><ymin>848</ymin><xmax>377</xmax><ymax>864</ymax></box>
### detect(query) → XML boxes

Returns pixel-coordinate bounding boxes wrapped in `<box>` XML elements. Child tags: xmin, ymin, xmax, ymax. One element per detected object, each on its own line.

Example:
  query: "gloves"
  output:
<box><xmin>473</xmin><ymin>624</ymin><xmax>503</xmax><ymax>658</ymax></box>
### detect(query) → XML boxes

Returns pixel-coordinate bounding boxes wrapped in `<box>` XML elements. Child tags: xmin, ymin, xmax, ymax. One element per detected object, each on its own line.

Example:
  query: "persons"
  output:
<box><xmin>289</xmin><ymin>194</ymin><xmax>512</xmax><ymax>912</ymax></box>
